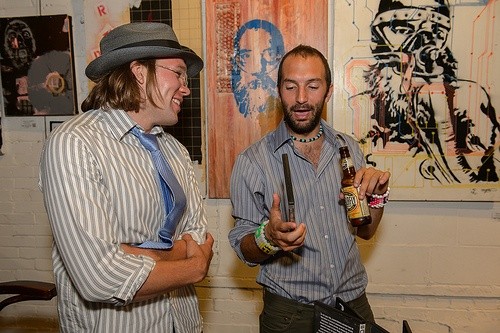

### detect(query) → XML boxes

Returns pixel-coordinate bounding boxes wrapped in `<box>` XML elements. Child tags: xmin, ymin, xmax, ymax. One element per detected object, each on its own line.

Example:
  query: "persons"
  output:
<box><xmin>229</xmin><ymin>45</ymin><xmax>393</xmax><ymax>333</ymax></box>
<box><xmin>38</xmin><ymin>21</ymin><xmax>214</xmax><ymax>333</ymax></box>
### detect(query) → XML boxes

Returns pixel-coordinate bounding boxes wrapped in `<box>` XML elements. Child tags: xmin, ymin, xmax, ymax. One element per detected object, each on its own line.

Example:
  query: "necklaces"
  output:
<box><xmin>288</xmin><ymin>122</ymin><xmax>323</xmax><ymax>142</ymax></box>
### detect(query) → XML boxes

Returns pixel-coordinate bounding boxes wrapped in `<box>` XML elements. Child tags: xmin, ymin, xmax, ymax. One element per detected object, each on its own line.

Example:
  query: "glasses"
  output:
<box><xmin>156</xmin><ymin>64</ymin><xmax>188</xmax><ymax>86</ymax></box>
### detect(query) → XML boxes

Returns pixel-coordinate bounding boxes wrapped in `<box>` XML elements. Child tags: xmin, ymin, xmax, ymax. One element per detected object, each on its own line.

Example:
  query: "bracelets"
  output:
<box><xmin>367</xmin><ymin>186</ymin><xmax>390</xmax><ymax>208</ymax></box>
<box><xmin>253</xmin><ymin>220</ymin><xmax>280</xmax><ymax>256</ymax></box>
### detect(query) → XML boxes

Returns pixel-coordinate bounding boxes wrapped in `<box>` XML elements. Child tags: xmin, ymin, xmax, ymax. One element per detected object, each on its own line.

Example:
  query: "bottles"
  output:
<box><xmin>339</xmin><ymin>145</ymin><xmax>372</xmax><ymax>228</ymax></box>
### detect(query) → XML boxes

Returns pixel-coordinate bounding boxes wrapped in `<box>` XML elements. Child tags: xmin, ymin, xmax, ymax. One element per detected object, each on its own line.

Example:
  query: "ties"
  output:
<box><xmin>130</xmin><ymin>127</ymin><xmax>187</xmax><ymax>250</ymax></box>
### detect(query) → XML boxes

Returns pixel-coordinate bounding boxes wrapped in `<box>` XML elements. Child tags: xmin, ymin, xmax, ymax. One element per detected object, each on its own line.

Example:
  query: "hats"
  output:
<box><xmin>84</xmin><ymin>21</ymin><xmax>204</xmax><ymax>83</ymax></box>
